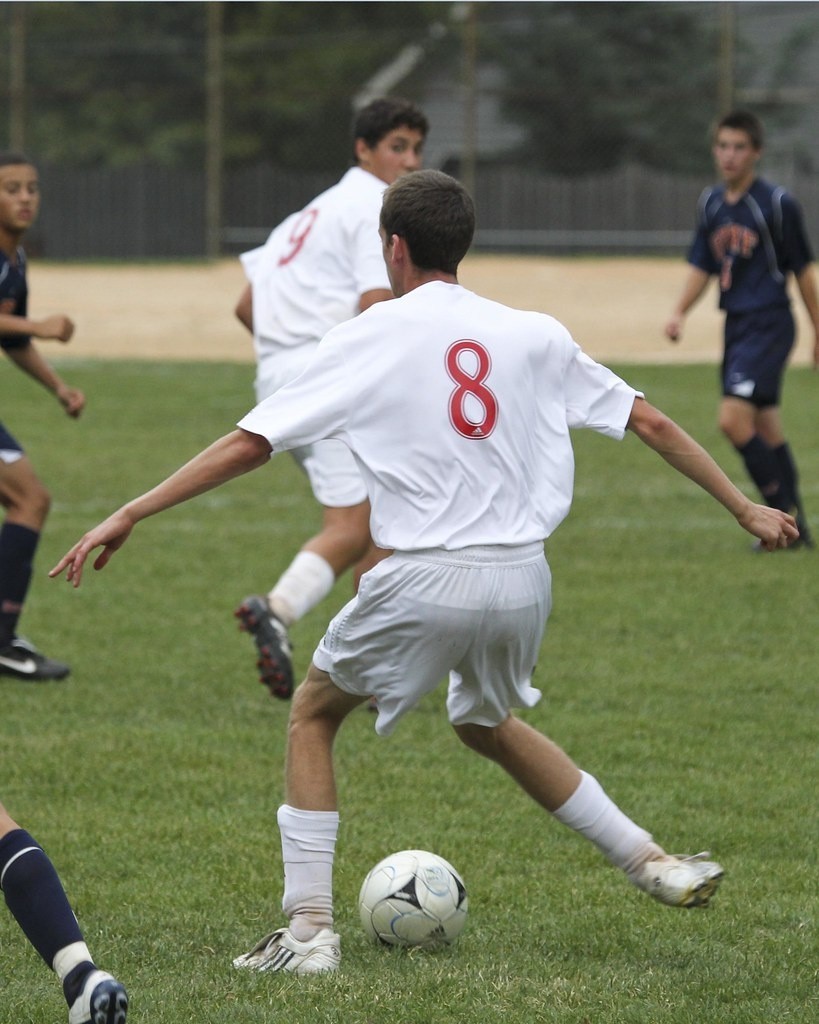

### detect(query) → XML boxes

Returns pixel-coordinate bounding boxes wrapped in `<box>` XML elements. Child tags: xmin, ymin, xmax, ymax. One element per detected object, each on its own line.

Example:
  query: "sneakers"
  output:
<box><xmin>0</xmin><ymin>637</ymin><xmax>72</xmax><ymax>682</ymax></box>
<box><xmin>69</xmin><ymin>970</ymin><xmax>129</xmax><ymax>1024</ymax></box>
<box><xmin>233</xmin><ymin>595</ymin><xmax>295</xmax><ymax>701</ymax></box>
<box><xmin>635</xmin><ymin>851</ymin><xmax>724</xmax><ymax>908</ymax></box>
<box><xmin>232</xmin><ymin>927</ymin><xmax>341</xmax><ymax>972</ymax></box>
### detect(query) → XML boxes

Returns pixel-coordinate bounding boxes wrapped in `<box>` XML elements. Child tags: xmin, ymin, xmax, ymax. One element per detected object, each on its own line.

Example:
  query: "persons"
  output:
<box><xmin>0</xmin><ymin>97</ymin><xmax>819</xmax><ymax>1024</ymax></box>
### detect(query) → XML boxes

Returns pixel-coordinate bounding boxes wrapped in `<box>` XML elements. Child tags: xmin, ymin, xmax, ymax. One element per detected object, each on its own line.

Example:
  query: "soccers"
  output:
<box><xmin>358</xmin><ymin>850</ymin><xmax>470</xmax><ymax>950</ymax></box>
